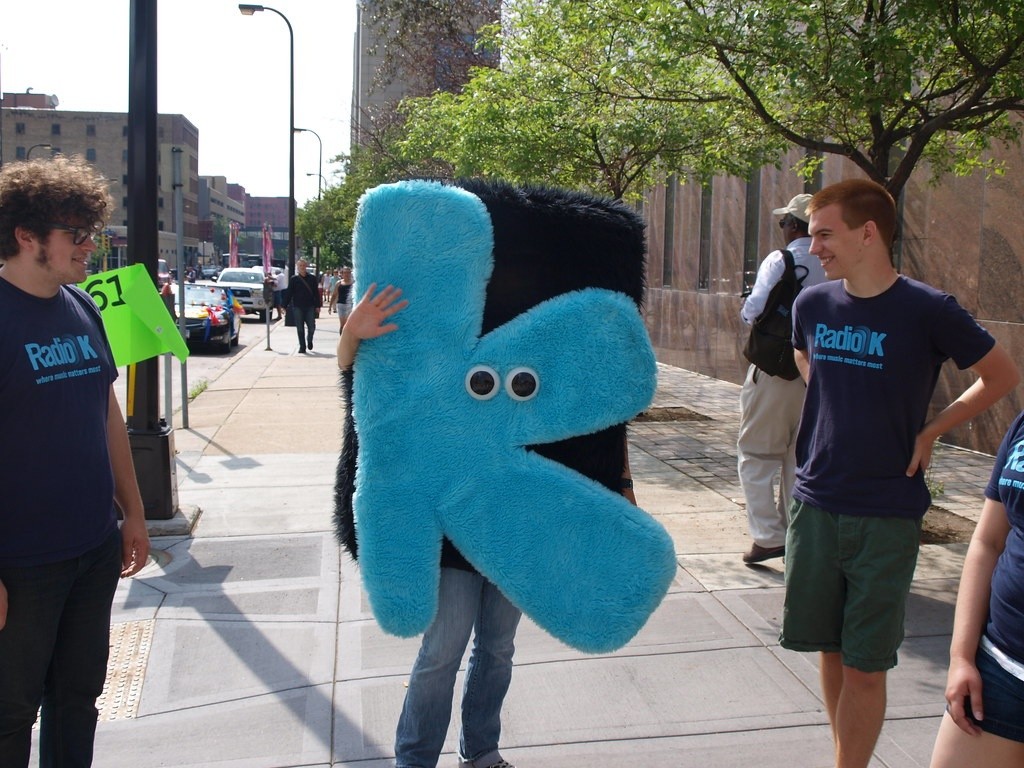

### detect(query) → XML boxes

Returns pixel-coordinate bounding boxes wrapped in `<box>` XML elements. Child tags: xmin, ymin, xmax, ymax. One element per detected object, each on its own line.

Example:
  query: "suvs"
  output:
<box><xmin>157</xmin><ymin>258</ymin><xmax>172</xmax><ymax>288</ymax></box>
<box><xmin>212</xmin><ymin>267</ymin><xmax>274</xmax><ymax>323</ymax></box>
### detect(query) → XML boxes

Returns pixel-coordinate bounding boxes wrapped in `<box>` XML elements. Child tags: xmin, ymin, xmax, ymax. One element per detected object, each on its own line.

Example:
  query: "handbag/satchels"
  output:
<box><xmin>313</xmin><ymin>309</ymin><xmax>319</xmax><ymax>319</ymax></box>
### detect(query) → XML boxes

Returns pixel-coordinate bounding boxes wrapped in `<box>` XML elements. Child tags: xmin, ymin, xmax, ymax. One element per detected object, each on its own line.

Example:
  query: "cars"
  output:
<box><xmin>306</xmin><ymin>267</ymin><xmax>320</xmax><ymax>279</ymax></box>
<box><xmin>165</xmin><ymin>281</ymin><xmax>240</xmax><ymax>352</ymax></box>
<box><xmin>200</xmin><ymin>265</ymin><xmax>221</xmax><ymax>279</ymax></box>
<box><xmin>253</xmin><ymin>265</ymin><xmax>282</xmax><ymax>277</ymax></box>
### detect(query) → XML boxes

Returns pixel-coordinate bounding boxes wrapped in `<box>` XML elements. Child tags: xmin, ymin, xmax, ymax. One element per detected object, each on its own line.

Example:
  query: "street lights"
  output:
<box><xmin>307</xmin><ymin>174</ymin><xmax>328</xmax><ymax>192</ymax></box>
<box><xmin>295</xmin><ymin>128</ymin><xmax>323</xmax><ymax>281</ymax></box>
<box><xmin>239</xmin><ymin>3</ymin><xmax>295</xmax><ymax>287</ymax></box>
<box><xmin>26</xmin><ymin>143</ymin><xmax>53</xmax><ymax>160</ymax></box>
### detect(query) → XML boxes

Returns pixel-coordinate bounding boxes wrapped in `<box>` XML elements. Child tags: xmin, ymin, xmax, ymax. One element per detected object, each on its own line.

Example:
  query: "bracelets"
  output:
<box><xmin>622</xmin><ymin>478</ymin><xmax>633</xmax><ymax>489</ymax></box>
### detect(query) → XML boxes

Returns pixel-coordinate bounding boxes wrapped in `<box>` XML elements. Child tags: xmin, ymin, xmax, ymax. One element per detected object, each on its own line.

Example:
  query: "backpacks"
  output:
<box><xmin>740</xmin><ymin>248</ymin><xmax>810</xmax><ymax>385</ymax></box>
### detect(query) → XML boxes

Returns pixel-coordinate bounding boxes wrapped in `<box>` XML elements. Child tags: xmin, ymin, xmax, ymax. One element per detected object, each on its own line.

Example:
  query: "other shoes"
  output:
<box><xmin>307</xmin><ymin>337</ymin><xmax>314</xmax><ymax>350</ymax></box>
<box><xmin>743</xmin><ymin>543</ymin><xmax>786</xmax><ymax>563</ymax></box>
<box><xmin>488</xmin><ymin>761</ymin><xmax>516</xmax><ymax>768</ymax></box>
<box><xmin>298</xmin><ymin>349</ymin><xmax>306</xmax><ymax>353</ymax></box>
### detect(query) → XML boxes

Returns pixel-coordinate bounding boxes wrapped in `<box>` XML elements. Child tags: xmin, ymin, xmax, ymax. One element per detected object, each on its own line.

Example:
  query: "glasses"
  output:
<box><xmin>778</xmin><ymin>217</ymin><xmax>795</xmax><ymax>228</ymax></box>
<box><xmin>50</xmin><ymin>223</ymin><xmax>95</xmax><ymax>246</ymax></box>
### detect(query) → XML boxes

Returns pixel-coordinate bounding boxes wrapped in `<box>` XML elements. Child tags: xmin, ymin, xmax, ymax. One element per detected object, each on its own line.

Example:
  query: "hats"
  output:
<box><xmin>772</xmin><ymin>194</ymin><xmax>812</xmax><ymax>223</ymax></box>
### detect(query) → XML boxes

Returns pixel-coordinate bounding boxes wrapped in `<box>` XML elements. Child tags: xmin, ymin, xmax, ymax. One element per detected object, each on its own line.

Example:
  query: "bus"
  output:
<box><xmin>222</xmin><ymin>255</ymin><xmax>285</xmax><ymax>269</ymax></box>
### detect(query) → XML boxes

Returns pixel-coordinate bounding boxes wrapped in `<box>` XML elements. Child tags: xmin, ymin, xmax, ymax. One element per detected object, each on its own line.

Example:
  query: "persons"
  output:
<box><xmin>336</xmin><ymin>283</ymin><xmax>635</xmax><ymax>768</ymax></box>
<box><xmin>175</xmin><ymin>262</ymin><xmax>204</xmax><ymax>284</ymax></box>
<box><xmin>0</xmin><ymin>155</ymin><xmax>152</xmax><ymax>768</ymax></box>
<box><xmin>930</xmin><ymin>413</ymin><xmax>1024</xmax><ymax>768</ymax></box>
<box><xmin>778</xmin><ymin>179</ymin><xmax>1022</xmax><ymax>768</ymax></box>
<box><xmin>263</xmin><ymin>259</ymin><xmax>356</xmax><ymax>354</ymax></box>
<box><xmin>740</xmin><ymin>193</ymin><xmax>832</xmax><ymax>564</ymax></box>
